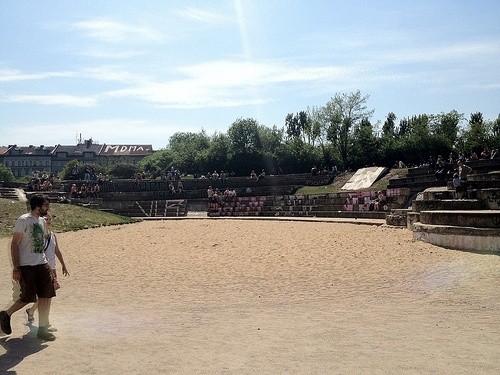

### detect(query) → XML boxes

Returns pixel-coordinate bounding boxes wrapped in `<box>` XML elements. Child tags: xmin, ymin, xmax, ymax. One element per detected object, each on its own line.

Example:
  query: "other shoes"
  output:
<box><xmin>48</xmin><ymin>324</ymin><xmax>57</xmax><ymax>332</ymax></box>
<box><xmin>37</xmin><ymin>327</ymin><xmax>56</xmax><ymax>341</ymax></box>
<box><xmin>25</xmin><ymin>308</ymin><xmax>34</xmax><ymax>322</ymax></box>
<box><xmin>0</xmin><ymin>311</ymin><xmax>12</xmax><ymax>335</ymax></box>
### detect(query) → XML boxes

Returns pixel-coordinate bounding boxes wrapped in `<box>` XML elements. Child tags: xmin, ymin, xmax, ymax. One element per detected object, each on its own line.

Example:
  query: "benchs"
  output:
<box><xmin>24</xmin><ymin>157</ymin><xmax>500</xmax><ymax>253</ymax></box>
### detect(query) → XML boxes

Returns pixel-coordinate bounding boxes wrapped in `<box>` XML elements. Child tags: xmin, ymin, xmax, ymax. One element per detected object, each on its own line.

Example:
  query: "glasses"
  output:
<box><xmin>43</xmin><ymin>203</ymin><xmax>49</xmax><ymax>208</ymax></box>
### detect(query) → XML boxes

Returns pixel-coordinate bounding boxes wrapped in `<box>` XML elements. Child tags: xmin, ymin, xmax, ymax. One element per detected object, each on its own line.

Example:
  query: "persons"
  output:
<box><xmin>0</xmin><ymin>192</ymin><xmax>61</xmax><ymax>344</ymax></box>
<box><xmin>24</xmin><ymin>211</ymin><xmax>72</xmax><ymax>335</ymax></box>
<box><xmin>29</xmin><ymin>144</ymin><xmax>500</xmax><ymax>216</ymax></box>
